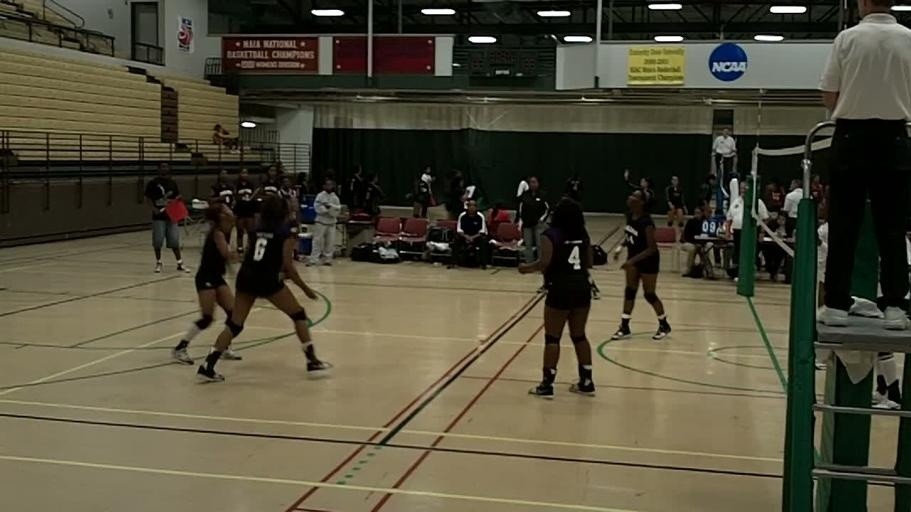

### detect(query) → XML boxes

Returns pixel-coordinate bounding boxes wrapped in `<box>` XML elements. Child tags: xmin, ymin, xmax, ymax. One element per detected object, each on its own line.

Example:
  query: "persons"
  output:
<box><xmin>170</xmin><ymin>203</ymin><xmax>242</xmax><ymax>366</ymax></box>
<box><xmin>141</xmin><ymin>159</ymin><xmax>655</xmax><ymax>277</ymax></box>
<box><xmin>527</xmin><ymin>196</ymin><xmax>597</xmax><ymax>399</ymax></box>
<box><xmin>213</xmin><ymin>124</ymin><xmax>240</xmax><ymax>154</ymax></box>
<box><xmin>195</xmin><ymin>197</ymin><xmax>335</xmax><ymax>382</ymax></box>
<box><xmin>712</xmin><ymin>127</ymin><xmax>736</xmax><ymax>173</ymax></box>
<box><xmin>611</xmin><ymin>190</ymin><xmax>671</xmax><ymax>341</ymax></box>
<box><xmin>816</xmin><ymin>1</ymin><xmax>911</xmax><ymax>330</ymax></box>
<box><xmin>664</xmin><ymin>176</ymin><xmax>904</xmax><ymax>410</ymax></box>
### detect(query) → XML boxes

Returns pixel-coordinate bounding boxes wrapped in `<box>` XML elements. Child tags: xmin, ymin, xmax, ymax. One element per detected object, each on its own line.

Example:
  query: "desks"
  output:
<box><xmin>178</xmin><ymin>201</ymin><xmax>212</xmax><ymax>250</ymax></box>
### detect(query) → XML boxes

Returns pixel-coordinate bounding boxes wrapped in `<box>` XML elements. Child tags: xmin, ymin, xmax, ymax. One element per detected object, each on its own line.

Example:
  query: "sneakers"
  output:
<box><xmin>171</xmin><ymin>345</ymin><xmax>333</xmax><ymax>382</ymax></box>
<box><xmin>154</xmin><ymin>262</ymin><xmax>162</xmax><ymax>272</ymax></box>
<box><xmin>872</xmin><ymin>391</ymin><xmax>888</xmax><ymax>403</ymax></box>
<box><xmin>528</xmin><ymin>325</ymin><xmax>671</xmax><ymax>398</ymax></box>
<box><xmin>884</xmin><ymin>306</ymin><xmax>910</xmax><ymax>329</ymax></box>
<box><xmin>818</xmin><ymin>304</ymin><xmax>848</xmax><ymax>325</ymax></box>
<box><xmin>177</xmin><ymin>263</ymin><xmax>190</xmax><ymax>273</ymax></box>
<box><xmin>872</xmin><ymin>399</ymin><xmax>901</xmax><ymax>410</ymax></box>
